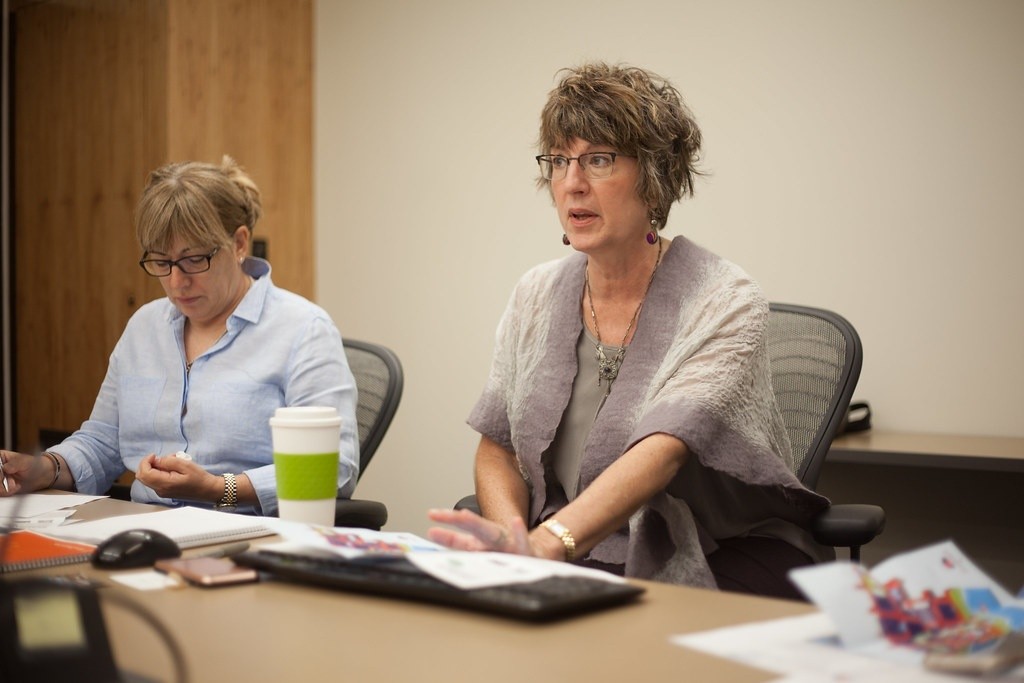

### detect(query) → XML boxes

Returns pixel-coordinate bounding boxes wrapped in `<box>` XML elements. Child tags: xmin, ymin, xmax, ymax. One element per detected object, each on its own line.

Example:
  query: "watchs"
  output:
<box><xmin>214</xmin><ymin>473</ymin><xmax>238</xmax><ymax>512</ymax></box>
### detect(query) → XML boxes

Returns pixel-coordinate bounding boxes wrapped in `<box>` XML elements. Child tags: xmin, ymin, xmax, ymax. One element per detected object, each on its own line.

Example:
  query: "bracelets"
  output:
<box><xmin>542</xmin><ymin>518</ymin><xmax>577</xmax><ymax>561</ymax></box>
<box><xmin>43</xmin><ymin>452</ymin><xmax>60</xmax><ymax>489</ymax></box>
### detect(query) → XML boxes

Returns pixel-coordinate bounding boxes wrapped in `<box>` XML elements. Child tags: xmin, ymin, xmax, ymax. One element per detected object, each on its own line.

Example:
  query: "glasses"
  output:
<box><xmin>139</xmin><ymin>248</ymin><xmax>221</xmax><ymax>277</ymax></box>
<box><xmin>535</xmin><ymin>151</ymin><xmax>637</xmax><ymax>181</ymax></box>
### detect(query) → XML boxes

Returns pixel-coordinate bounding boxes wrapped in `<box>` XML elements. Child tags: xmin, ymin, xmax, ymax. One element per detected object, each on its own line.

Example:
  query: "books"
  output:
<box><xmin>0</xmin><ymin>505</ymin><xmax>278</xmax><ymax>573</ymax></box>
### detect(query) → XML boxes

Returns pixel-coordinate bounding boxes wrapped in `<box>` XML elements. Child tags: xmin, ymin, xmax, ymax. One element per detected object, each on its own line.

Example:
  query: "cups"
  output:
<box><xmin>269</xmin><ymin>407</ymin><xmax>343</xmax><ymax>528</ymax></box>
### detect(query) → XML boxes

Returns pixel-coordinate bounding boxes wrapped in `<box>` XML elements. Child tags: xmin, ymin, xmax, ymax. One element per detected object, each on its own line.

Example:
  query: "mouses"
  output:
<box><xmin>90</xmin><ymin>528</ymin><xmax>182</xmax><ymax>570</ymax></box>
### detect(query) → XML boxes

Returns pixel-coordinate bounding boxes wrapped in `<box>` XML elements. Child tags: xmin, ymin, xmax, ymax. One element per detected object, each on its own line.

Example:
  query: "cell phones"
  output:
<box><xmin>155</xmin><ymin>557</ymin><xmax>257</xmax><ymax>585</ymax></box>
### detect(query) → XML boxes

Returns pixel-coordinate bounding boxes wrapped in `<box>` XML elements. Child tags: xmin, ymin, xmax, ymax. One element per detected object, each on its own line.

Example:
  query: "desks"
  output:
<box><xmin>0</xmin><ymin>490</ymin><xmax>823</xmax><ymax>683</ymax></box>
<box><xmin>825</xmin><ymin>424</ymin><xmax>1024</xmax><ymax>473</ymax></box>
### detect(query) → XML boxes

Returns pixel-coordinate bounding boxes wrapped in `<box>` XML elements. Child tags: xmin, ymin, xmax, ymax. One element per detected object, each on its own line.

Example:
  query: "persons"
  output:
<box><xmin>0</xmin><ymin>161</ymin><xmax>359</xmax><ymax>517</ymax></box>
<box><xmin>428</xmin><ymin>66</ymin><xmax>831</xmax><ymax>604</ymax></box>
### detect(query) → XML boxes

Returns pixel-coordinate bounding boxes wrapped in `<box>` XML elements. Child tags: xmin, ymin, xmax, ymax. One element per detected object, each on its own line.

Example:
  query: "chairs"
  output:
<box><xmin>334</xmin><ymin>337</ymin><xmax>404</xmax><ymax>532</ymax></box>
<box><xmin>705</xmin><ymin>300</ymin><xmax>887</xmax><ymax>599</ymax></box>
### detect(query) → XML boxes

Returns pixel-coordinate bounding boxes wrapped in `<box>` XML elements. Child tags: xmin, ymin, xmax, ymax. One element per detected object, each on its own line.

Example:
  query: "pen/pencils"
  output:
<box><xmin>0</xmin><ymin>449</ymin><xmax>10</xmax><ymax>494</ymax></box>
<box><xmin>199</xmin><ymin>542</ymin><xmax>247</xmax><ymax>557</ymax></box>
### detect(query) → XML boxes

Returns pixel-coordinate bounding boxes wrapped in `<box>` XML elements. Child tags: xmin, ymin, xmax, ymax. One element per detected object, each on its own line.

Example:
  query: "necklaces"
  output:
<box><xmin>585</xmin><ymin>238</ymin><xmax>662</xmax><ymax>397</ymax></box>
